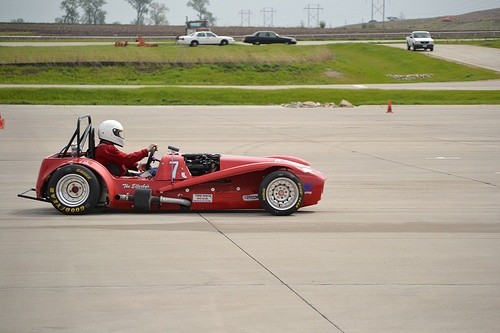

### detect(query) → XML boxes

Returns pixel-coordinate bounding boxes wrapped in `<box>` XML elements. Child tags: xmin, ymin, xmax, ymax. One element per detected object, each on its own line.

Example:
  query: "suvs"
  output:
<box><xmin>185</xmin><ymin>19</ymin><xmax>210</xmax><ymax>36</ymax></box>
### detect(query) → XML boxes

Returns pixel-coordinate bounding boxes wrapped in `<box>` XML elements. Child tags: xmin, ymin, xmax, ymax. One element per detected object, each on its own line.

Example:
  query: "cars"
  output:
<box><xmin>242</xmin><ymin>31</ymin><xmax>297</xmax><ymax>45</ymax></box>
<box><xmin>178</xmin><ymin>30</ymin><xmax>236</xmax><ymax>47</ymax></box>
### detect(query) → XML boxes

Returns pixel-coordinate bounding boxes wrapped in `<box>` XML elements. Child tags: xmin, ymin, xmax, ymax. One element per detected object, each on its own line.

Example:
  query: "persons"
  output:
<box><xmin>95</xmin><ymin>120</ymin><xmax>158</xmax><ymax>176</ymax></box>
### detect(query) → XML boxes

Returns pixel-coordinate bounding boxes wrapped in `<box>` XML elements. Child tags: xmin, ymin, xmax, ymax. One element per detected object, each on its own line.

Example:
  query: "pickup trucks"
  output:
<box><xmin>406</xmin><ymin>31</ymin><xmax>435</xmax><ymax>51</ymax></box>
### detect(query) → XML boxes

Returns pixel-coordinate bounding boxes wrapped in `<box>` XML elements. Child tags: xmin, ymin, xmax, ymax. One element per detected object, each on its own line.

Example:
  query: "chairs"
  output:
<box><xmin>88</xmin><ymin>127</ymin><xmax>96</xmax><ymax>158</ymax></box>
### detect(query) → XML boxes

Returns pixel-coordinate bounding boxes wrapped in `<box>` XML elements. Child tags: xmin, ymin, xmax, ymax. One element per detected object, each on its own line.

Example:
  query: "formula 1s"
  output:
<box><xmin>17</xmin><ymin>115</ymin><xmax>328</xmax><ymax>217</ymax></box>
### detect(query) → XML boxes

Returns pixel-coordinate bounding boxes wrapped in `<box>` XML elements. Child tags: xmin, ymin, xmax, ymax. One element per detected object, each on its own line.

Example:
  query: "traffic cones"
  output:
<box><xmin>385</xmin><ymin>98</ymin><xmax>394</xmax><ymax>113</ymax></box>
<box><xmin>0</xmin><ymin>112</ymin><xmax>6</xmax><ymax>129</ymax></box>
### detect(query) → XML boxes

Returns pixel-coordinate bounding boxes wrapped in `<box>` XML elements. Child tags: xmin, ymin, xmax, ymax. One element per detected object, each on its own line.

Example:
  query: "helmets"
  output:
<box><xmin>98</xmin><ymin>120</ymin><xmax>125</xmax><ymax>147</ymax></box>
